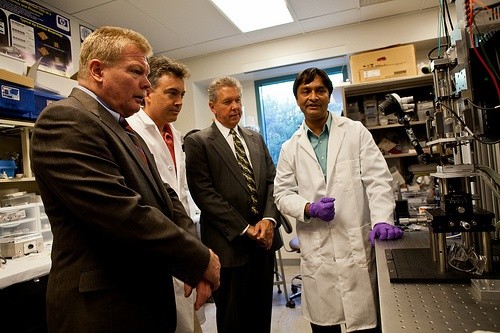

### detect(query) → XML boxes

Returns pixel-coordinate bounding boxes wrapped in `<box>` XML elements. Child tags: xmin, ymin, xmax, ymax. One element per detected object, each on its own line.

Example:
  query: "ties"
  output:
<box><xmin>231</xmin><ymin>128</ymin><xmax>259</xmax><ymax>215</ymax></box>
<box><xmin>119</xmin><ymin>116</ymin><xmax>149</xmax><ymax>167</ymax></box>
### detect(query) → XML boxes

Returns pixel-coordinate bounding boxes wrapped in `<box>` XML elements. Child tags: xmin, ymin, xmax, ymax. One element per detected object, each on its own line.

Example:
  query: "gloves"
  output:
<box><xmin>309</xmin><ymin>197</ymin><xmax>335</xmax><ymax>221</ymax></box>
<box><xmin>370</xmin><ymin>223</ymin><xmax>403</xmax><ymax>245</ymax></box>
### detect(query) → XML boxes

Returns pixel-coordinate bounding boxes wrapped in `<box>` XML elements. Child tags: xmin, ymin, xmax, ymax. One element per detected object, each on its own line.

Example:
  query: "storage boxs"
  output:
<box><xmin>390</xmin><ymin>166</ymin><xmax>405</xmax><ymax>186</ymax></box>
<box><xmin>0</xmin><ymin>160</ymin><xmax>18</xmax><ymax>177</ymax></box>
<box><xmin>347</xmin><ymin>43</ymin><xmax>417</xmax><ymax>84</ymax></box>
<box><xmin>0</xmin><ymin>195</ymin><xmax>53</xmax><ymax>256</ymax></box>
<box><xmin>0</xmin><ymin>52</ymin><xmax>68</xmax><ymax>121</ymax></box>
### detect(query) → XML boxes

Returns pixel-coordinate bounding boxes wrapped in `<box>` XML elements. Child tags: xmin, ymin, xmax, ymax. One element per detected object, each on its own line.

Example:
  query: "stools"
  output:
<box><xmin>289</xmin><ymin>236</ymin><xmax>301</xmax><ymax>308</ymax></box>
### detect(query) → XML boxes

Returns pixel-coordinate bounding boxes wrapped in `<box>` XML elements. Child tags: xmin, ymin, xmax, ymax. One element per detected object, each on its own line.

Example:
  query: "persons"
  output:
<box><xmin>125</xmin><ymin>55</ymin><xmax>210</xmax><ymax>332</ymax></box>
<box><xmin>30</xmin><ymin>26</ymin><xmax>220</xmax><ymax>332</ymax></box>
<box><xmin>273</xmin><ymin>66</ymin><xmax>402</xmax><ymax>333</ymax></box>
<box><xmin>183</xmin><ymin>76</ymin><xmax>284</xmax><ymax>333</ymax></box>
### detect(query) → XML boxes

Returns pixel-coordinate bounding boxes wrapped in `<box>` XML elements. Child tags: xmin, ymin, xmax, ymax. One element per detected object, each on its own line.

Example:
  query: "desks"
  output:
<box><xmin>0</xmin><ymin>251</ymin><xmax>52</xmax><ymax>289</ymax></box>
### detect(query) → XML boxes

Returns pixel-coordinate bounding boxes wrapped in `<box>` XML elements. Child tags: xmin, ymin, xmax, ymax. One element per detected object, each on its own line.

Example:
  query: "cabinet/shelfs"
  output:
<box><xmin>342</xmin><ymin>75</ymin><xmax>455</xmax><ymax>201</ymax></box>
